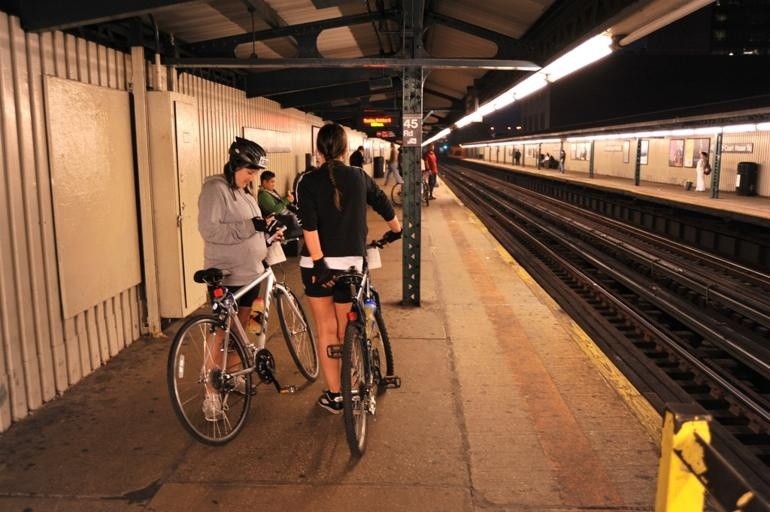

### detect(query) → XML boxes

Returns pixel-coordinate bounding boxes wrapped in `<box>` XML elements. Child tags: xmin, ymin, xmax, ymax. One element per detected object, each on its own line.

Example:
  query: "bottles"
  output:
<box><xmin>364</xmin><ymin>294</ymin><xmax>376</xmax><ymax>339</ymax></box>
<box><xmin>247</xmin><ymin>296</ymin><xmax>265</xmax><ymax>337</ymax></box>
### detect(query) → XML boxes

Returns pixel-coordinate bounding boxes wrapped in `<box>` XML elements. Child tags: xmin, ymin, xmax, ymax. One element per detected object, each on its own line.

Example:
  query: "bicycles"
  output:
<box><xmin>162</xmin><ymin>223</ymin><xmax>324</xmax><ymax>451</ymax></box>
<box><xmin>390</xmin><ymin>174</ymin><xmax>431</xmax><ymax>210</ymax></box>
<box><xmin>305</xmin><ymin>226</ymin><xmax>412</xmax><ymax>467</ymax></box>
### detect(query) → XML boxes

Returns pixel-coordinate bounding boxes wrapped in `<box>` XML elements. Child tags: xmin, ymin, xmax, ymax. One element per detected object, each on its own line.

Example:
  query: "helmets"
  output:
<box><xmin>228</xmin><ymin>135</ymin><xmax>269</xmax><ymax>170</ymax></box>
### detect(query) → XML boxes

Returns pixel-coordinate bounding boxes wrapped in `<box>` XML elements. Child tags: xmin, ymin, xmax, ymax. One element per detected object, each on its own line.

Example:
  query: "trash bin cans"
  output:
<box><xmin>374</xmin><ymin>157</ymin><xmax>384</xmax><ymax>178</ymax></box>
<box><xmin>736</xmin><ymin>162</ymin><xmax>759</xmax><ymax>196</ymax></box>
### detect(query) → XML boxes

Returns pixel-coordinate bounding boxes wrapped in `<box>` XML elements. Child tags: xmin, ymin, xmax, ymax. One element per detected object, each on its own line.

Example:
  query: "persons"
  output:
<box><xmin>695</xmin><ymin>152</ymin><xmax>707</xmax><ymax>191</ymax></box>
<box><xmin>514</xmin><ymin>149</ymin><xmax>521</xmax><ymax>166</ymax></box>
<box><xmin>540</xmin><ymin>153</ymin><xmax>551</xmax><ymax>163</ymax></box>
<box><xmin>294</xmin><ymin>124</ymin><xmax>402</xmax><ymax>415</ymax></box>
<box><xmin>425</xmin><ymin>143</ymin><xmax>438</xmax><ymax>198</ymax></box>
<box><xmin>257</xmin><ymin>170</ymin><xmax>302</xmax><ymax>227</ymax></box>
<box><xmin>198</xmin><ymin>137</ymin><xmax>283</xmax><ymax>421</ymax></box>
<box><xmin>559</xmin><ymin>149</ymin><xmax>566</xmax><ymax>173</ymax></box>
<box><xmin>383</xmin><ymin>142</ymin><xmax>402</xmax><ymax>185</ymax></box>
<box><xmin>350</xmin><ymin>146</ymin><xmax>364</xmax><ymax>168</ymax></box>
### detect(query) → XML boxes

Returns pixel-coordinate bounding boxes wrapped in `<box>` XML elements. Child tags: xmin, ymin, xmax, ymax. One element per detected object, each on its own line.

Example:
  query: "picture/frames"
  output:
<box><xmin>312</xmin><ymin>125</ymin><xmax>322</xmax><ymax>156</ymax></box>
<box><xmin>669</xmin><ymin>139</ymin><xmax>685</xmax><ymax>167</ymax></box>
<box><xmin>623</xmin><ymin>140</ymin><xmax>630</xmax><ymax>163</ymax></box>
<box><xmin>684</xmin><ymin>138</ymin><xmax>710</xmax><ymax>168</ymax></box>
<box><xmin>639</xmin><ymin>140</ymin><xmax>649</xmax><ymax>165</ymax></box>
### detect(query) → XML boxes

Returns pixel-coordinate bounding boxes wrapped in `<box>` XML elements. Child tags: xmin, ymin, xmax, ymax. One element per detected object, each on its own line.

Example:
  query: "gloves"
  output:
<box><xmin>251</xmin><ymin>216</ymin><xmax>268</xmax><ymax>231</ymax></box>
<box><xmin>383</xmin><ymin>228</ymin><xmax>402</xmax><ymax>243</ymax></box>
<box><xmin>311</xmin><ymin>256</ymin><xmax>333</xmax><ymax>286</ymax></box>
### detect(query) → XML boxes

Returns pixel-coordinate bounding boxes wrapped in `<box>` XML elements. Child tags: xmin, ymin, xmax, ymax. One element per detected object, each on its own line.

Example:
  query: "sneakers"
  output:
<box><xmin>318</xmin><ymin>389</ymin><xmax>344</xmax><ymax>416</ymax></box>
<box><xmin>204</xmin><ymin>392</ymin><xmax>222</xmax><ymax>423</ymax></box>
<box><xmin>429</xmin><ymin>195</ymin><xmax>436</xmax><ymax>200</ymax></box>
<box><xmin>224</xmin><ymin>376</ymin><xmax>257</xmax><ymax>396</ymax></box>
